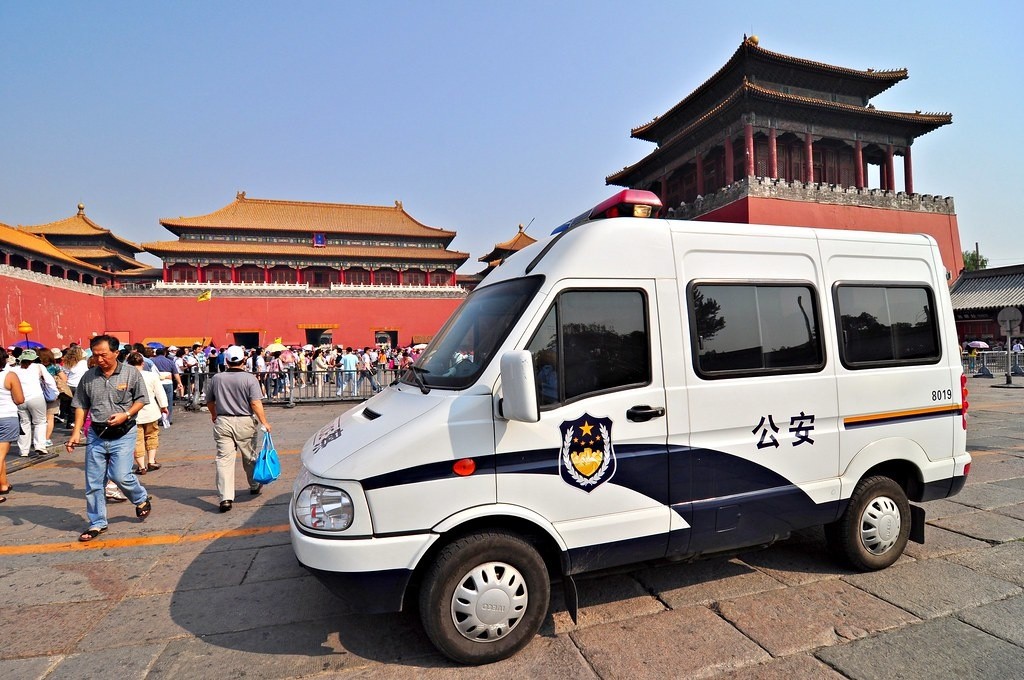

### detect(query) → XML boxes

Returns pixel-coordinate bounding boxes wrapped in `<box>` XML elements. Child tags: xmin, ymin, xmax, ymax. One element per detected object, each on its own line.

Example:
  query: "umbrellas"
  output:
<box><xmin>968</xmin><ymin>341</ymin><xmax>989</xmax><ymax>349</ymax></box>
<box><xmin>147</xmin><ymin>342</ymin><xmax>166</xmax><ymax>348</ymax></box>
<box><xmin>266</xmin><ymin>342</ymin><xmax>289</xmax><ymax>352</ymax></box>
<box><xmin>412</xmin><ymin>344</ymin><xmax>427</xmax><ymax>348</ymax></box>
<box><xmin>14</xmin><ymin>340</ymin><xmax>44</xmax><ymax>350</ymax></box>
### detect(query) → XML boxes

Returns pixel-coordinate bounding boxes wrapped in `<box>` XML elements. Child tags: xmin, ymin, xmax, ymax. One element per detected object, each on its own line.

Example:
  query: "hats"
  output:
<box><xmin>50</xmin><ymin>347</ymin><xmax>63</xmax><ymax>359</ymax></box>
<box><xmin>168</xmin><ymin>345</ymin><xmax>180</xmax><ymax>351</ymax></box>
<box><xmin>12</xmin><ymin>347</ymin><xmax>23</xmax><ymax>357</ymax></box>
<box><xmin>17</xmin><ymin>350</ymin><xmax>39</xmax><ymax>361</ymax></box>
<box><xmin>225</xmin><ymin>345</ymin><xmax>244</xmax><ymax>362</ymax></box>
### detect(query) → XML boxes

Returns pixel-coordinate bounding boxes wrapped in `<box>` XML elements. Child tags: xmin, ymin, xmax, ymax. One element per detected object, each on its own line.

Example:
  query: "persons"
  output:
<box><xmin>0</xmin><ymin>342</ymin><xmax>90</xmax><ymax>434</ymax></box>
<box><xmin>126</xmin><ymin>352</ymin><xmax>169</xmax><ymax>475</ymax></box>
<box><xmin>387</xmin><ymin>347</ymin><xmax>473</xmax><ymax>380</ymax></box>
<box><xmin>958</xmin><ymin>338</ymin><xmax>1024</xmax><ymax>373</ymax></box>
<box><xmin>205</xmin><ymin>346</ymin><xmax>272</xmax><ymax>512</ymax></box>
<box><xmin>308</xmin><ymin>346</ymin><xmax>388</xmax><ymax>396</ymax></box>
<box><xmin>240</xmin><ymin>345</ymin><xmax>308</xmax><ymax>402</ymax></box>
<box><xmin>36</xmin><ymin>347</ymin><xmax>67</xmax><ymax>447</ymax></box>
<box><xmin>83</xmin><ymin>342</ymin><xmax>161</xmax><ymax>438</ymax></box>
<box><xmin>166</xmin><ymin>341</ymin><xmax>235</xmax><ymax>401</ymax></box>
<box><xmin>11</xmin><ymin>349</ymin><xmax>60</xmax><ymax>457</ymax></box>
<box><xmin>149</xmin><ymin>348</ymin><xmax>183</xmax><ymax>425</ymax></box>
<box><xmin>0</xmin><ymin>346</ymin><xmax>25</xmax><ymax>502</ymax></box>
<box><xmin>67</xmin><ymin>334</ymin><xmax>152</xmax><ymax>540</ymax></box>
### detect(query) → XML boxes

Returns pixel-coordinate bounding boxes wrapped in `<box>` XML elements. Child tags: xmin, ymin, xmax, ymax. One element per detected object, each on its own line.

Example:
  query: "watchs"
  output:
<box><xmin>126</xmin><ymin>411</ymin><xmax>131</xmax><ymax>419</ymax></box>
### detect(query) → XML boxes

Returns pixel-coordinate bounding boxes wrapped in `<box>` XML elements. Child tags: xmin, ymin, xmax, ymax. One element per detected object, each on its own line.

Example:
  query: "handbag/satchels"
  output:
<box><xmin>280</xmin><ymin>371</ymin><xmax>286</xmax><ymax>379</ymax></box>
<box><xmin>54</xmin><ymin>363</ymin><xmax>67</xmax><ymax>383</ymax></box>
<box><xmin>38</xmin><ymin>364</ymin><xmax>56</xmax><ymax>400</ymax></box>
<box><xmin>252</xmin><ymin>432</ymin><xmax>281</xmax><ymax>484</ymax></box>
<box><xmin>92</xmin><ymin>418</ymin><xmax>136</xmax><ymax>441</ymax></box>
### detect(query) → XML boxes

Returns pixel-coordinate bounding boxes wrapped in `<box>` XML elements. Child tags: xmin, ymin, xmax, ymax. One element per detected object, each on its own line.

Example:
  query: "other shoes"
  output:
<box><xmin>148</xmin><ymin>461</ymin><xmax>161</xmax><ymax>470</ymax></box>
<box><xmin>53</xmin><ymin>417</ymin><xmax>65</xmax><ymax>423</ymax></box>
<box><xmin>105</xmin><ymin>485</ymin><xmax>127</xmax><ymax>499</ymax></box>
<box><xmin>251</xmin><ymin>483</ymin><xmax>263</xmax><ymax>495</ymax></box>
<box><xmin>35</xmin><ymin>448</ymin><xmax>48</xmax><ymax>456</ymax></box>
<box><xmin>65</xmin><ymin>425</ymin><xmax>74</xmax><ymax>429</ymax></box>
<box><xmin>136</xmin><ymin>466</ymin><xmax>147</xmax><ymax>474</ymax></box>
<box><xmin>219</xmin><ymin>500</ymin><xmax>233</xmax><ymax>512</ymax></box>
<box><xmin>46</xmin><ymin>439</ymin><xmax>54</xmax><ymax>446</ymax></box>
<box><xmin>21</xmin><ymin>453</ymin><xmax>28</xmax><ymax>456</ymax></box>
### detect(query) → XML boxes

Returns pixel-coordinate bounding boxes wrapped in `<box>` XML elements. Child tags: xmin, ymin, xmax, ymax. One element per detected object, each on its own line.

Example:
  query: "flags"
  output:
<box><xmin>196</xmin><ymin>290</ymin><xmax>210</xmax><ymax>302</ymax></box>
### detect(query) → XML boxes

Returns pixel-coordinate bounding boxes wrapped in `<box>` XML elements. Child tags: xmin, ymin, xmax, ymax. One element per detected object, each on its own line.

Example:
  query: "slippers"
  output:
<box><xmin>0</xmin><ymin>496</ymin><xmax>7</xmax><ymax>503</ymax></box>
<box><xmin>0</xmin><ymin>484</ymin><xmax>13</xmax><ymax>494</ymax></box>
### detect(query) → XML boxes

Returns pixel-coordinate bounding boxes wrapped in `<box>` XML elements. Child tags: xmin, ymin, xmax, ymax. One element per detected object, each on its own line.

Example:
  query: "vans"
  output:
<box><xmin>287</xmin><ymin>188</ymin><xmax>970</xmax><ymax>667</ymax></box>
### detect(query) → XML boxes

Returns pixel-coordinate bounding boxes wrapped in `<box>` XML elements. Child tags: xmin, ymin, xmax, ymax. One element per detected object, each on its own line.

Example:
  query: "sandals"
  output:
<box><xmin>136</xmin><ymin>499</ymin><xmax>151</xmax><ymax>519</ymax></box>
<box><xmin>81</xmin><ymin>526</ymin><xmax>108</xmax><ymax>541</ymax></box>
